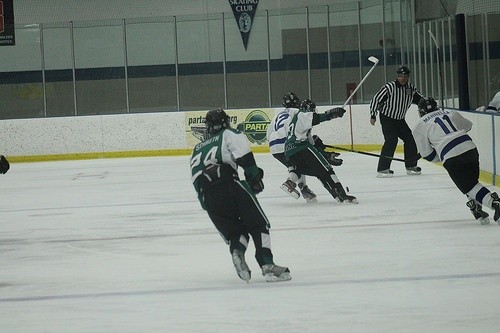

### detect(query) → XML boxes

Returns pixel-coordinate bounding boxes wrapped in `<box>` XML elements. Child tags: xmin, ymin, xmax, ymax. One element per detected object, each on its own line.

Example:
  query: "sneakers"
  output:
<box><xmin>232</xmin><ymin>249</ymin><xmax>252</xmax><ymax>284</ymax></box>
<box><xmin>377</xmin><ymin>169</ymin><xmax>394</xmax><ymax>177</ymax></box>
<box><xmin>280</xmin><ymin>180</ymin><xmax>300</xmax><ymax>199</ymax></box>
<box><xmin>487</xmin><ymin>193</ymin><xmax>500</xmax><ymax>225</ymax></box>
<box><xmin>298</xmin><ymin>183</ymin><xmax>317</xmax><ymax>203</ymax></box>
<box><xmin>337</xmin><ymin>195</ymin><xmax>359</xmax><ymax>204</ymax></box>
<box><xmin>405</xmin><ymin>161</ymin><xmax>421</xmax><ymax>175</ymax></box>
<box><xmin>260</xmin><ymin>264</ymin><xmax>291</xmax><ymax>282</ymax></box>
<box><xmin>466</xmin><ymin>200</ymin><xmax>490</xmax><ymax>226</ymax></box>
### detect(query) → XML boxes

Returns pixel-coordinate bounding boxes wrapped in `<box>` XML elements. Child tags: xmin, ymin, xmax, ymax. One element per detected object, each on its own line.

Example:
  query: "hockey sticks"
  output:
<box><xmin>323</xmin><ymin>145</ymin><xmax>422</xmax><ymax>163</ymax></box>
<box><xmin>341</xmin><ymin>56</ymin><xmax>380</xmax><ymax>108</ymax></box>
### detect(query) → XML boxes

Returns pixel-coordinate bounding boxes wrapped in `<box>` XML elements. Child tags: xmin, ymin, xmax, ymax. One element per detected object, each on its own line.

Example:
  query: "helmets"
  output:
<box><xmin>418</xmin><ymin>97</ymin><xmax>438</xmax><ymax>116</ymax></box>
<box><xmin>283</xmin><ymin>93</ymin><xmax>300</xmax><ymax>108</ymax></box>
<box><xmin>205</xmin><ymin>109</ymin><xmax>229</xmax><ymax>132</ymax></box>
<box><xmin>397</xmin><ymin>66</ymin><xmax>410</xmax><ymax>75</ymax></box>
<box><xmin>300</xmin><ymin>99</ymin><xmax>315</xmax><ymax>112</ymax></box>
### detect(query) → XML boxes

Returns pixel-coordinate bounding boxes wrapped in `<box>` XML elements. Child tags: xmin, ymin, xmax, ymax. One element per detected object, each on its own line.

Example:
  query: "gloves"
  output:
<box><xmin>326</xmin><ymin>151</ymin><xmax>343</xmax><ymax>166</ymax></box>
<box><xmin>246</xmin><ymin>174</ymin><xmax>264</xmax><ymax>193</ymax></box>
<box><xmin>325</xmin><ymin>107</ymin><xmax>346</xmax><ymax>119</ymax></box>
<box><xmin>312</xmin><ymin>134</ymin><xmax>327</xmax><ymax>150</ymax></box>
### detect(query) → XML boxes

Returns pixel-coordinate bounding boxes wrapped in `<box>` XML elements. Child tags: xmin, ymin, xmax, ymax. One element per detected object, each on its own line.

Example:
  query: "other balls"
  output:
<box><xmin>346</xmin><ymin>187</ymin><xmax>349</xmax><ymax>192</ymax></box>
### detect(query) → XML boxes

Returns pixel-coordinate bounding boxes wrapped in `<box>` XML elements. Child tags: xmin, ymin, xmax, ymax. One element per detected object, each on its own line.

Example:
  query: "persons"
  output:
<box><xmin>370</xmin><ymin>66</ymin><xmax>423</xmax><ymax>174</ymax></box>
<box><xmin>0</xmin><ymin>154</ymin><xmax>10</xmax><ymax>175</ymax></box>
<box><xmin>410</xmin><ymin>96</ymin><xmax>500</xmax><ymax>222</ymax></box>
<box><xmin>189</xmin><ymin>108</ymin><xmax>290</xmax><ymax>281</ymax></box>
<box><xmin>266</xmin><ymin>91</ymin><xmax>357</xmax><ymax>203</ymax></box>
